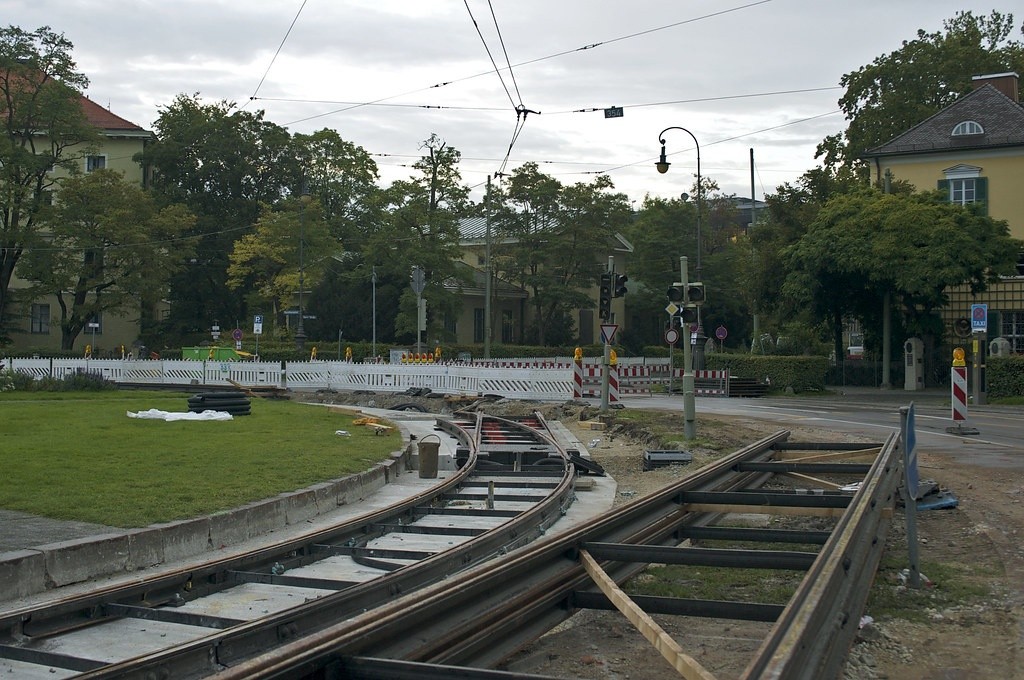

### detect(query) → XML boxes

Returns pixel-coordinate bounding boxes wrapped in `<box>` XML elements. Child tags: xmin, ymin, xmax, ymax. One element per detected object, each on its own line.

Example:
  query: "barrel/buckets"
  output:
<box><xmin>417</xmin><ymin>434</ymin><xmax>441</xmax><ymax>479</ymax></box>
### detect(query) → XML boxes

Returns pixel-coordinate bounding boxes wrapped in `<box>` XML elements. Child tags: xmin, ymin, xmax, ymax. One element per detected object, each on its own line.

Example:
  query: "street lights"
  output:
<box><xmin>653</xmin><ymin>126</ymin><xmax>703</xmax><ymax>327</ymax></box>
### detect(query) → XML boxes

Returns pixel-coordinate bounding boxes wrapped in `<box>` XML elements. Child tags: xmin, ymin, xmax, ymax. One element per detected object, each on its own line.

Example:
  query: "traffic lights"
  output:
<box><xmin>680</xmin><ymin>307</ymin><xmax>698</xmax><ymax>325</ymax></box>
<box><xmin>666</xmin><ymin>285</ymin><xmax>684</xmax><ymax>301</ymax></box>
<box><xmin>599</xmin><ymin>273</ymin><xmax>611</xmax><ymax>321</ymax></box>
<box><xmin>614</xmin><ymin>274</ymin><xmax>628</xmax><ymax>298</ymax></box>
<box><xmin>687</xmin><ymin>283</ymin><xmax>705</xmax><ymax>303</ymax></box>
